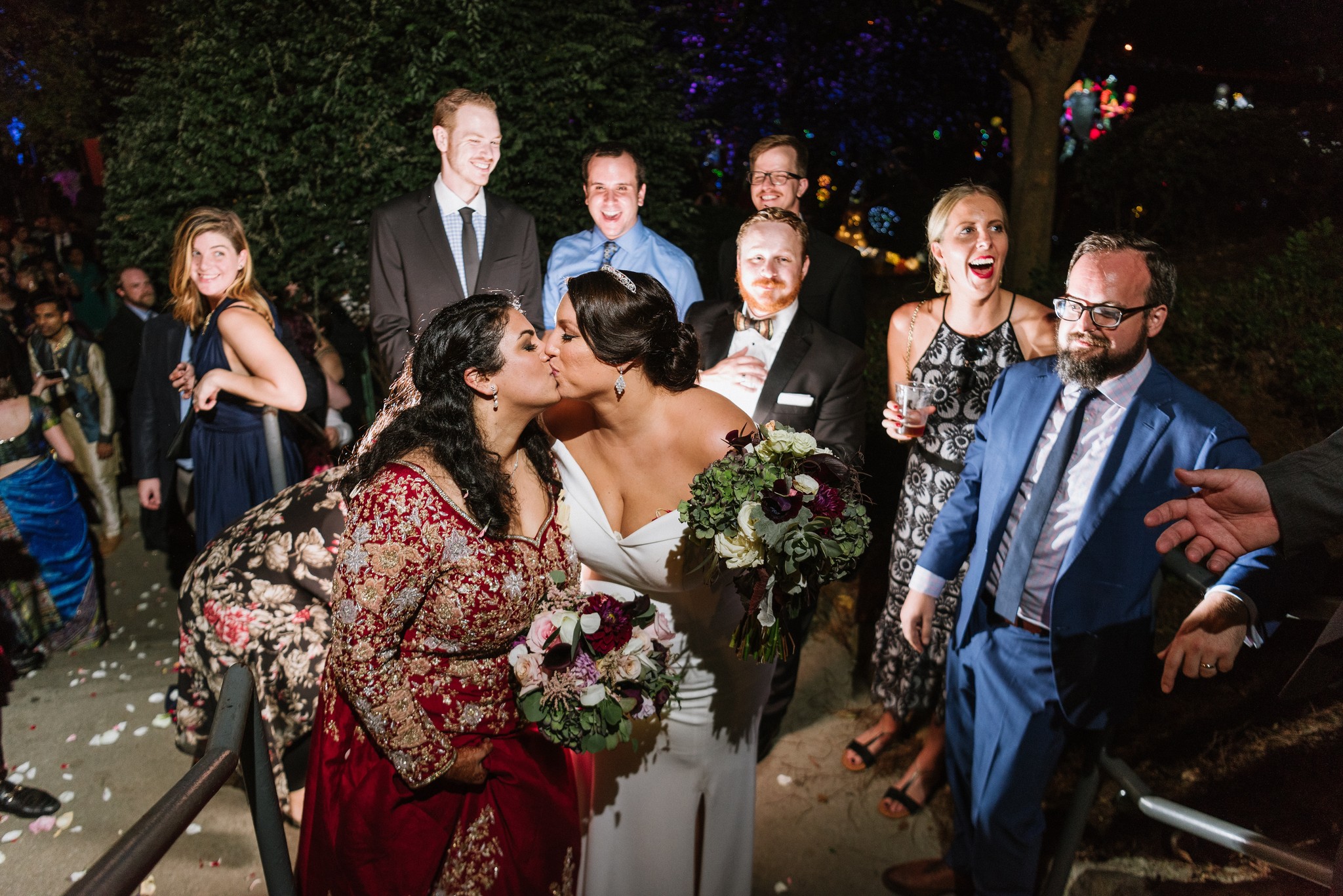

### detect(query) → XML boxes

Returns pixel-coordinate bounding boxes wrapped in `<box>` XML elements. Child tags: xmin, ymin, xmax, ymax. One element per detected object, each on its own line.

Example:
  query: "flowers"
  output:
<box><xmin>508</xmin><ymin>569</ymin><xmax>682</xmax><ymax>755</ymax></box>
<box><xmin>677</xmin><ymin>421</ymin><xmax>878</xmax><ymax>663</ymax></box>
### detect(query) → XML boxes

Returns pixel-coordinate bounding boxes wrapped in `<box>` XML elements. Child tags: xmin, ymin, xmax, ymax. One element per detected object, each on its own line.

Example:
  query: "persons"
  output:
<box><xmin>675</xmin><ymin>204</ymin><xmax>868</xmax><ymax>781</ymax></box>
<box><xmin>322</xmin><ymin>409</ymin><xmax>357</xmax><ymax>453</ymax></box>
<box><xmin>365</xmin><ymin>84</ymin><xmax>549</xmax><ymax>406</ymax></box>
<box><xmin>0</xmin><ymin>161</ymin><xmax>123</xmax><ymax>823</ymax></box>
<box><xmin>1143</xmin><ymin>417</ymin><xmax>1342</xmax><ymax>577</ymax></box>
<box><xmin>880</xmin><ymin>230</ymin><xmax>1303</xmax><ymax>895</ymax></box>
<box><xmin>531</xmin><ymin>262</ymin><xmax>790</xmax><ymax>896</ymax></box>
<box><xmin>173</xmin><ymin>455</ymin><xmax>349</xmax><ymax>828</ymax></box>
<box><xmin>249</xmin><ymin>242</ymin><xmax>330</xmax><ymax>482</ymax></box>
<box><xmin>287</xmin><ymin>283</ymin><xmax>592</xmax><ymax>896</ymax></box>
<box><xmin>132</xmin><ymin>305</ymin><xmax>211</xmax><ymax>612</ymax></box>
<box><xmin>541</xmin><ymin>136</ymin><xmax>702</xmax><ymax>354</ymax></box>
<box><xmin>104</xmin><ymin>260</ymin><xmax>178</xmax><ymax>484</ymax></box>
<box><xmin>716</xmin><ymin>129</ymin><xmax>864</xmax><ymax>358</ymax></box>
<box><xmin>166</xmin><ymin>208</ymin><xmax>307</xmax><ymax>592</ymax></box>
<box><xmin>280</xmin><ymin>274</ymin><xmax>346</xmax><ymax>385</ymax></box>
<box><xmin>840</xmin><ymin>179</ymin><xmax>1063</xmax><ymax>825</ymax></box>
<box><xmin>324</xmin><ymin>374</ymin><xmax>358</xmax><ymax>412</ymax></box>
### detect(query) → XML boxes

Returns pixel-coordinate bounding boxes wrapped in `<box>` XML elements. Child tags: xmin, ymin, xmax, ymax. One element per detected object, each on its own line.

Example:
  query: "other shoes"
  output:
<box><xmin>99</xmin><ymin>533</ymin><xmax>121</xmax><ymax>556</ymax></box>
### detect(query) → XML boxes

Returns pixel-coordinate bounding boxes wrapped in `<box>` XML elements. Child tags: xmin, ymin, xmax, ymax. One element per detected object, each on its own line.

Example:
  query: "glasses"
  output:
<box><xmin>1053</xmin><ymin>295</ymin><xmax>1157</xmax><ymax>327</ymax></box>
<box><xmin>746</xmin><ymin>170</ymin><xmax>802</xmax><ymax>186</ymax></box>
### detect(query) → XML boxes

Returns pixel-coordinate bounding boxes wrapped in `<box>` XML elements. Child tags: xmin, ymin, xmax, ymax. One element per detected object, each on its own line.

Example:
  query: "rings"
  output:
<box><xmin>741</xmin><ymin>373</ymin><xmax>746</xmax><ymax>383</ymax></box>
<box><xmin>1200</xmin><ymin>661</ymin><xmax>1216</xmax><ymax>669</ymax></box>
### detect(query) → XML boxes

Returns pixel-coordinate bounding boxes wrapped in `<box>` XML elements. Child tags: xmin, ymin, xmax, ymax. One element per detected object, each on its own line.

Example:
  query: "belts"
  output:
<box><xmin>978</xmin><ymin>587</ymin><xmax>1047</xmax><ymax>637</ymax></box>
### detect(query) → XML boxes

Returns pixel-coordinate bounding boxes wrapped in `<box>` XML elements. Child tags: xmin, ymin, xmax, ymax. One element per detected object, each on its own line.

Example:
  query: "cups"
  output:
<box><xmin>895</xmin><ymin>379</ymin><xmax>937</xmax><ymax>438</ymax></box>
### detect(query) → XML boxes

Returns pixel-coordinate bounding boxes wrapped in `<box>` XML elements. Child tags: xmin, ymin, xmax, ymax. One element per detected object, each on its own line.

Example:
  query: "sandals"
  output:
<box><xmin>878</xmin><ymin>759</ymin><xmax>945</xmax><ymax>819</ymax></box>
<box><xmin>839</xmin><ymin>722</ymin><xmax>902</xmax><ymax>771</ymax></box>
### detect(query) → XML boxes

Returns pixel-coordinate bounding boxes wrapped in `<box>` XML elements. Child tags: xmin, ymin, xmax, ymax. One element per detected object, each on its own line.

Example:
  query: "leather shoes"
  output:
<box><xmin>0</xmin><ymin>780</ymin><xmax>62</xmax><ymax>817</ymax></box>
<box><xmin>882</xmin><ymin>857</ymin><xmax>957</xmax><ymax>892</ymax></box>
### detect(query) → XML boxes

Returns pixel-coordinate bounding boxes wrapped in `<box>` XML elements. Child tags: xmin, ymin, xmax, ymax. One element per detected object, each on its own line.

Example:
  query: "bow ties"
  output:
<box><xmin>731</xmin><ymin>310</ymin><xmax>773</xmax><ymax>340</ymax></box>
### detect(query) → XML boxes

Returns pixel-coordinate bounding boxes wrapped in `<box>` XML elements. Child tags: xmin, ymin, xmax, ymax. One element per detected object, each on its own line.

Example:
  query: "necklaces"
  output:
<box><xmin>499</xmin><ymin>436</ymin><xmax>520</xmax><ymax>496</ymax></box>
<box><xmin>200</xmin><ymin>311</ymin><xmax>212</xmax><ymax>335</ymax></box>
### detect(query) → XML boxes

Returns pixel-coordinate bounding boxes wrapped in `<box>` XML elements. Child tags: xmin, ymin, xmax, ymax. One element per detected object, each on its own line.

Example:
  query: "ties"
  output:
<box><xmin>456</xmin><ymin>207</ymin><xmax>481</xmax><ymax>300</ymax></box>
<box><xmin>59</xmin><ymin>234</ymin><xmax>64</xmax><ymax>257</ymax></box>
<box><xmin>596</xmin><ymin>241</ymin><xmax>621</xmax><ymax>273</ymax></box>
<box><xmin>993</xmin><ymin>387</ymin><xmax>1101</xmax><ymax>623</ymax></box>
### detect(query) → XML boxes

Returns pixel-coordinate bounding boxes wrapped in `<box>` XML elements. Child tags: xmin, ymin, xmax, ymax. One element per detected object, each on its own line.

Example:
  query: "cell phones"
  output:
<box><xmin>37</xmin><ymin>368</ymin><xmax>70</xmax><ymax>382</ymax></box>
